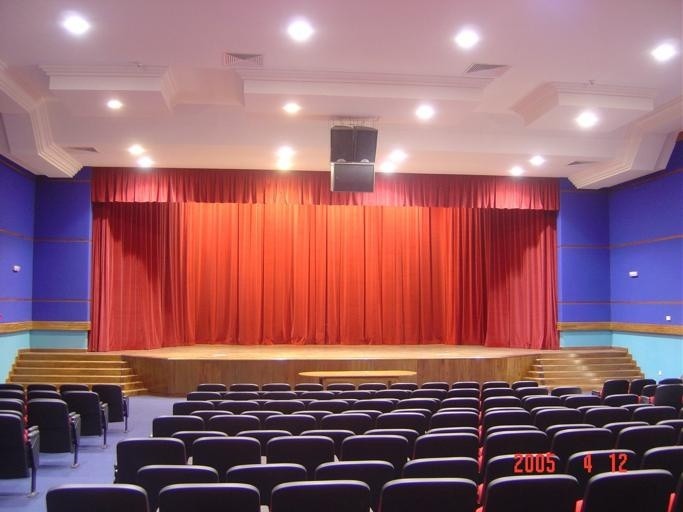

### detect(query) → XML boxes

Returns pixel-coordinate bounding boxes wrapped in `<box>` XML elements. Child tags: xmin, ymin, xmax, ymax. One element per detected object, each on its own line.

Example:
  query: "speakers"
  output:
<box><xmin>331</xmin><ymin>126</ymin><xmax>354</xmax><ymax>162</ymax></box>
<box><xmin>330</xmin><ymin>163</ymin><xmax>375</xmax><ymax>192</ymax></box>
<box><xmin>354</xmin><ymin>126</ymin><xmax>378</xmax><ymax>163</ymax></box>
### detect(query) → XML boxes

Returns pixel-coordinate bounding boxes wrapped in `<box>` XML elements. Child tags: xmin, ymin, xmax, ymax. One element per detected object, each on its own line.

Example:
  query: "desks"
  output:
<box><xmin>298</xmin><ymin>370</ymin><xmax>418</xmax><ymax>389</ymax></box>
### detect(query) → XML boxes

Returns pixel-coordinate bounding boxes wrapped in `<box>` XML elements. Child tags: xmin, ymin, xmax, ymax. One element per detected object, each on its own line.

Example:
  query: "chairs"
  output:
<box><xmin>0</xmin><ymin>378</ymin><xmax>683</xmax><ymax>512</ymax></box>
